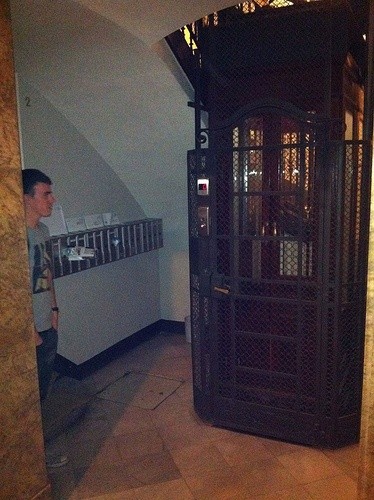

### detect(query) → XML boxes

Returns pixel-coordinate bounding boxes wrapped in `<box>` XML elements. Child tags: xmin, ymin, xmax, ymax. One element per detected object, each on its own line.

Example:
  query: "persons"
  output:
<box><xmin>20</xmin><ymin>168</ymin><xmax>70</xmax><ymax>468</ymax></box>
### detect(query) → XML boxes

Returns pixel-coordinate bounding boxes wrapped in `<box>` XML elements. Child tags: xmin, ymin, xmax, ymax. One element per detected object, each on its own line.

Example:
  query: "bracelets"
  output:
<box><xmin>51</xmin><ymin>307</ymin><xmax>59</xmax><ymax>311</ymax></box>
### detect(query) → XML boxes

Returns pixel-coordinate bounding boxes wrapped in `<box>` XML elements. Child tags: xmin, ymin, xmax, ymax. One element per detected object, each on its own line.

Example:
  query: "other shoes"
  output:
<box><xmin>46</xmin><ymin>455</ymin><xmax>68</xmax><ymax>468</ymax></box>
